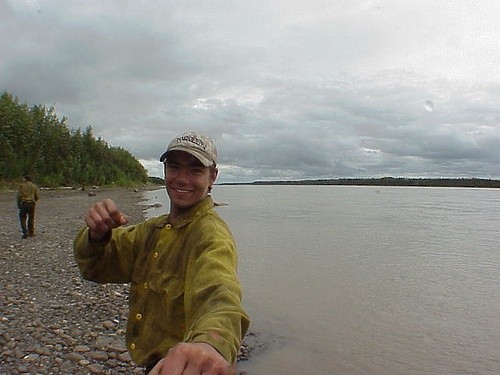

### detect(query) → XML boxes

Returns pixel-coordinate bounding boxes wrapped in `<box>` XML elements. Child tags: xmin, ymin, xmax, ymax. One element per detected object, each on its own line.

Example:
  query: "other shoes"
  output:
<box><xmin>22</xmin><ymin>232</ymin><xmax>27</xmax><ymax>239</ymax></box>
<box><xmin>30</xmin><ymin>232</ymin><xmax>34</xmax><ymax>236</ymax></box>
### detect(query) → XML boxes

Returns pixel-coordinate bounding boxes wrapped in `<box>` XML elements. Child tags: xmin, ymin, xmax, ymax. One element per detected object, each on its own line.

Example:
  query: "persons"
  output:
<box><xmin>16</xmin><ymin>175</ymin><xmax>39</xmax><ymax>239</ymax></box>
<box><xmin>73</xmin><ymin>130</ymin><xmax>251</xmax><ymax>375</ymax></box>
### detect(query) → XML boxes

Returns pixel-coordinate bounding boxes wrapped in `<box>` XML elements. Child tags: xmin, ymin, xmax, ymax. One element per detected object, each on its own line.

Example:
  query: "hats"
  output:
<box><xmin>159</xmin><ymin>131</ymin><xmax>218</xmax><ymax>167</ymax></box>
